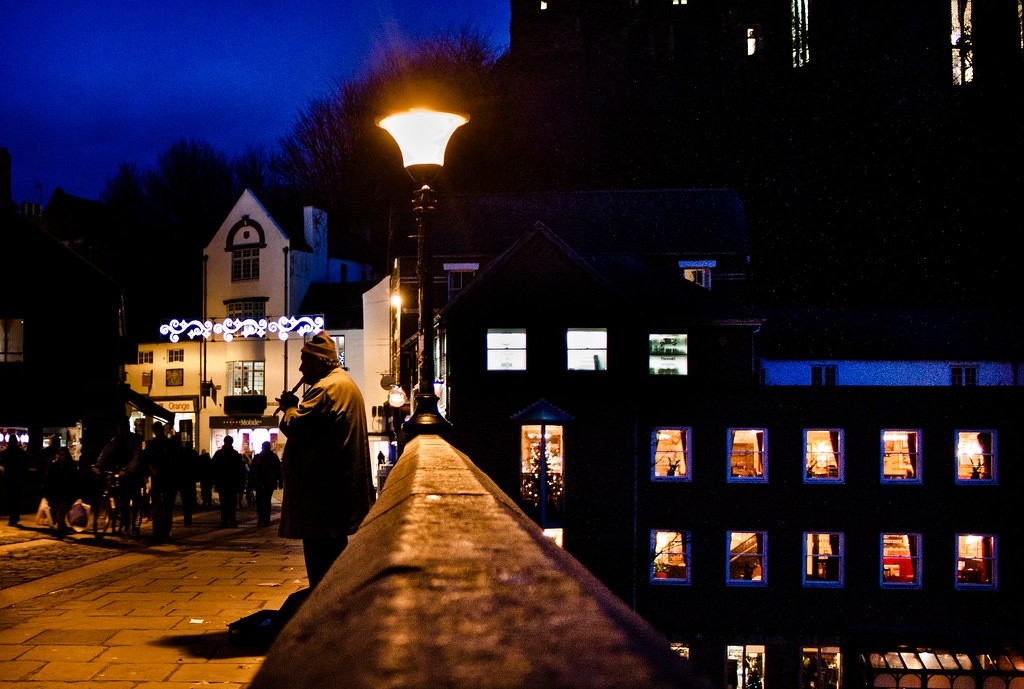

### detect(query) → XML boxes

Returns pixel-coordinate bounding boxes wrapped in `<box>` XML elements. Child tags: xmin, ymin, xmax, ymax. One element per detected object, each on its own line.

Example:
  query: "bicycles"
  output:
<box><xmin>93</xmin><ymin>467</ymin><xmax>142</xmax><ymax>541</ymax></box>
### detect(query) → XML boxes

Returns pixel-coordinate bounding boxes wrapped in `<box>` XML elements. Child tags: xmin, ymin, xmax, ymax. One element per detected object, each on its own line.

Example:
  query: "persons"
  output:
<box><xmin>277</xmin><ymin>332</ymin><xmax>377</xmax><ymax>590</ymax></box>
<box><xmin>0</xmin><ymin>421</ymin><xmax>281</xmax><ymax>543</ymax></box>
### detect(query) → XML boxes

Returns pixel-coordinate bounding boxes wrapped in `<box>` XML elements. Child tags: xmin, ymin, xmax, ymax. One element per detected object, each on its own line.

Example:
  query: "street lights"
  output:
<box><xmin>375</xmin><ymin>83</ymin><xmax>471</xmax><ymax>433</ymax></box>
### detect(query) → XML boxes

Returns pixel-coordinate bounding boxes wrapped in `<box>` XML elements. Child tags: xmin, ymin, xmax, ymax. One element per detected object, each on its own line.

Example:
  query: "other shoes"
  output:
<box><xmin>51</xmin><ymin>513</ymin><xmax>71</xmax><ymax>530</ymax></box>
<box><xmin>202</xmin><ymin>499</ymin><xmax>212</xmax><ymax>503</ymax></box>
<box><xmin>184</xmin><ymin>517</ymin><xmax>191</xmax><ymax>526</ymax></box>
<box><xmin>153</xmin><ymin>529</ymin><xmax>171</xmax><ymax>543</ymax></box>
<box><xmin>8</xmin><ymin>517</ymin><xmax>20</xmax><ymax>525</ymax></box>
<box><xmin>257</xmin><ymin>520</ymin><xmax>269</xmax><ymax>528</ymax></box>
<box><xmin>218</xmin><ymin>517</ymin><xmax>237</xmax><ymax>529</ymax></box>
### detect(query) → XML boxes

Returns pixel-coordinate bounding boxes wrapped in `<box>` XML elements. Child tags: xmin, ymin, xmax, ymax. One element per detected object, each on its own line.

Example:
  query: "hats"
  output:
<box><xmin>263</xmin><ymin>442</ymin><xmax>271</xmax><ymax>449</ymax></box>
<box><xmin>225</xmin><ymin>436</ymin><xmax>232</xmax><ymax>444</ymax></box>
<box><xmin>300</xmin><ymin>332</ymin><xmax>336</xmax><ymax>364</ymax></box>
<box><xmin>152</xmin><ymin>422</ymin><xmax>164</xmax><ymax>431</ymax></box>
